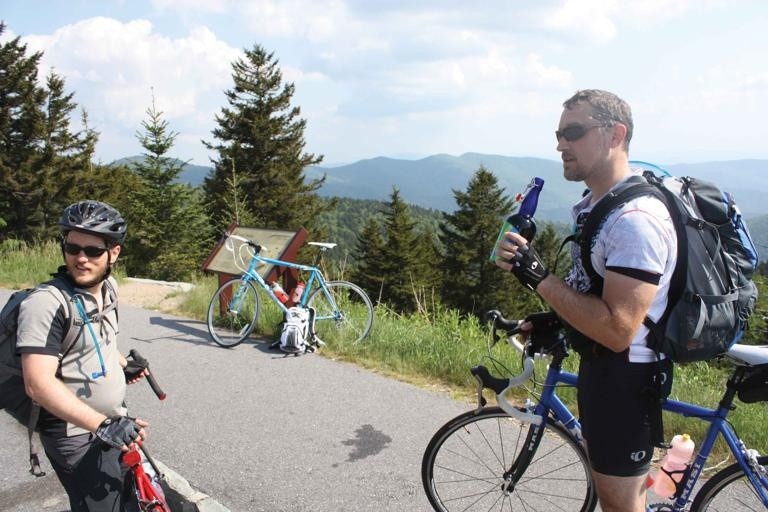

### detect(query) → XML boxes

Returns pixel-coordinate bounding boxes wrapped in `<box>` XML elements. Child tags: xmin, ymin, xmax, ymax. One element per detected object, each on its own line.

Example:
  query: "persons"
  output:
<box><xmin>14</xmin><ymin>199</ymin><xmax>152</xmax><ymax>511</ymax></box>
<box><xmin>494</xmin><ymin>89</ymin><xmax>681</xmax><ymax>512</ymax></box>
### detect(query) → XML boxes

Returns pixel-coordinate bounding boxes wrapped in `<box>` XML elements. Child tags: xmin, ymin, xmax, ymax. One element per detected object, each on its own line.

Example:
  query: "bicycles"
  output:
<box><xmin>206</xmin><ymin>232</ymin><xmax>373</xmax><ymax>350</ymax></box>
<box><xmin>422</xmin><ymin>308</ymin><xmax>768</xmax><ymax>510</ymax></box>
<box><xmin>114</xmin><ymin>350</ymin><xmax>170</xmax><ymax>511</ymax></box>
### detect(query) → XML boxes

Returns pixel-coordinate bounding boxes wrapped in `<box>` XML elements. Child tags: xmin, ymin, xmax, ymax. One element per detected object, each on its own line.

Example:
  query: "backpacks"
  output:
<box><xmin>0</xmin><ymin>278</ymin><xmax>118</xmax><ymax>433</ymax></box>
<box><xmin>579</xmin><ymin>171</ymin><xmax>758</xmax><ymax>364</ymax></box>
<box><xmin>280</xmin><ymin>306</ymin><xmax>326</xmax><ymax>354</ymax></box>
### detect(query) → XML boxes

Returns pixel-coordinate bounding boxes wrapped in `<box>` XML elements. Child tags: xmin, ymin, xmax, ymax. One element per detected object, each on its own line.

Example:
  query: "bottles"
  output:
<box><xmin>653</xmin><ymin>433</ymin><xmax>697</xmax><ymax>499</ymax></box>
<box><xmin>488</xmin><ymin>176</ymin><xmax>546</xmax><ymax>270</ymax></box>
<box><xmin>291</xmin><ymin>280</ymin><xmax>306</xmax><ymax>304</ymax></box>
<box><xmin>272</xmin><ymin>281</ymin><xmax>289</xmax><ymax>304</ymax></box>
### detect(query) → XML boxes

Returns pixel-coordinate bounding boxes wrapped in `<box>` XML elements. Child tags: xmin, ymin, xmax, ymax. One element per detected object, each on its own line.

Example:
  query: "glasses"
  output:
<box><xmin>555</xmin><ymin>121</ymin><xmax>615</xmax><ymax>141</ymax></box>
<box><xmin>59</xmin><ymin>234</ymin><xmax>116</xmax><ymax>259</ymax></box>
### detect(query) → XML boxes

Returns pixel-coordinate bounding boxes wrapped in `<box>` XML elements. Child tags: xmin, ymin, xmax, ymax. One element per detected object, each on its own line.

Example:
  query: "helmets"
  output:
<box><xmin>59</xmin><ymin>200</ymin><xmax>127</xmax><ymax>246</ymax></box>
